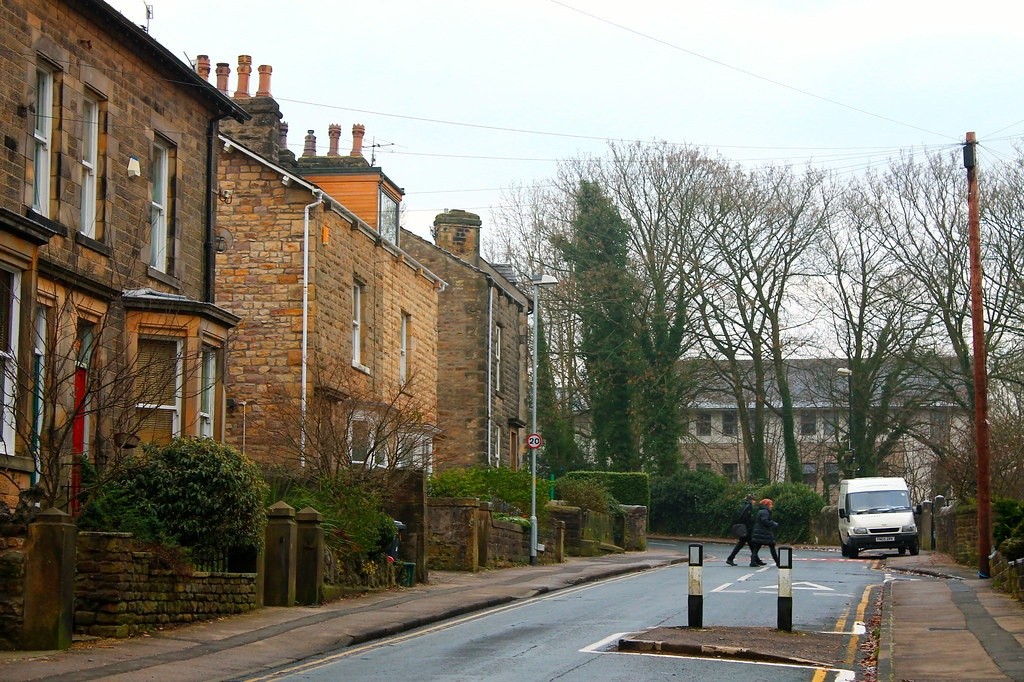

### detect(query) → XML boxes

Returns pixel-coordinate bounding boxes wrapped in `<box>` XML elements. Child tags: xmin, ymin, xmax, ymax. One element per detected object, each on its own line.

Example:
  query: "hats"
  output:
<box><xmin>760</xmin><ymin>498</ymin><xmax>773</xmax><ymax>507</ymax></box>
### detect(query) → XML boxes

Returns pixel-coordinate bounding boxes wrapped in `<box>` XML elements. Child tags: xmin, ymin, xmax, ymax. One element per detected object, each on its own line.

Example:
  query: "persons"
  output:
<box><xmin>749</xmin><ymin>499</ymin><xmax>779</xmax><ymax>567</ymax></box>
<box><xmin>726</xmin><ymin>493</ymin><xmax>767</xmax><ymax>565</ymax></box>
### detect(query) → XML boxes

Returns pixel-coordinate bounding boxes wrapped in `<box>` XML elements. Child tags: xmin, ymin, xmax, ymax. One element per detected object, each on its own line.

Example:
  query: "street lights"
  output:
<box><xmin>838</xmin><ymin>368</ymin><xmax>854</xmax><ymax>478</ymax></box>
<box><xmin>530</xmin><ymin>274</ymin><xmax>559</xmax><ymax>560</ymax></box>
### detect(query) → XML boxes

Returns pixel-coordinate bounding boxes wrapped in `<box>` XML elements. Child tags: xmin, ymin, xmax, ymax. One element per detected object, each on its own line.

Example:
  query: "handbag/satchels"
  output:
<box><xmin>732</xmin><ymin>525</ymin><xmax>748</xmax><ymax>537</ymax></box>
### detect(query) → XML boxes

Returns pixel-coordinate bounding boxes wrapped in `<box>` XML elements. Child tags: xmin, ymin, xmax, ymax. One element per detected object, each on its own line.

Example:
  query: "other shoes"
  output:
<box><xmin>750</xmin><ymin>563</ymin><xmax>760</xmax><ymax>568</ymax></box>
<box><xmin>726</xmin><ymin>559</ymin><xmax>738</xmax><ymax>566</ymax></box>
<box><xmin>757</xmin><ymin>560</ymin><xmax>767</xmax><ymax>565</ymax></box>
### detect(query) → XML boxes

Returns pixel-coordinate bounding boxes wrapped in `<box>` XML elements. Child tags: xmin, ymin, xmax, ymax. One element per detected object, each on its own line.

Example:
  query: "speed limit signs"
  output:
<box><xmin>527</xmin><ymin>434</ymin><xmax>542</xmax><ymax>448</ymax></box>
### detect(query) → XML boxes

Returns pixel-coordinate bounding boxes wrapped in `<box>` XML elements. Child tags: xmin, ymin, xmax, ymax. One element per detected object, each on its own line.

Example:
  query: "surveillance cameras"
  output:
<box><xmin>239</xmin><ymin>401</ymin><xmax>247</xmax><ymax>406</ymax></box>
<box><xmin>223</xmin><ymin>189</ymin><xmax>234</xmax><ymax>198</ymax></box>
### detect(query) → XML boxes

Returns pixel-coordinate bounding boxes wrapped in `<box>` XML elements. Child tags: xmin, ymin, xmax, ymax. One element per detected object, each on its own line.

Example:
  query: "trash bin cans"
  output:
<box><xmin>386</xmin><ymin>520</ymin><xmax>407</xmax><ymax>564</ymax></box>
<box><xmin>621</xmin><ymin>504</ymin><xmax>647</xmax><ymax>551</ymax></box>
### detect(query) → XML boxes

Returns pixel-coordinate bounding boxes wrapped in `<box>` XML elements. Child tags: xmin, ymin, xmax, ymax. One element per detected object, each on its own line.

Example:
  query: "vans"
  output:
<box><xmin>837</xmin><ymin>477</ymin><xmax>922</xmax><ymax>559</ymax></box>
